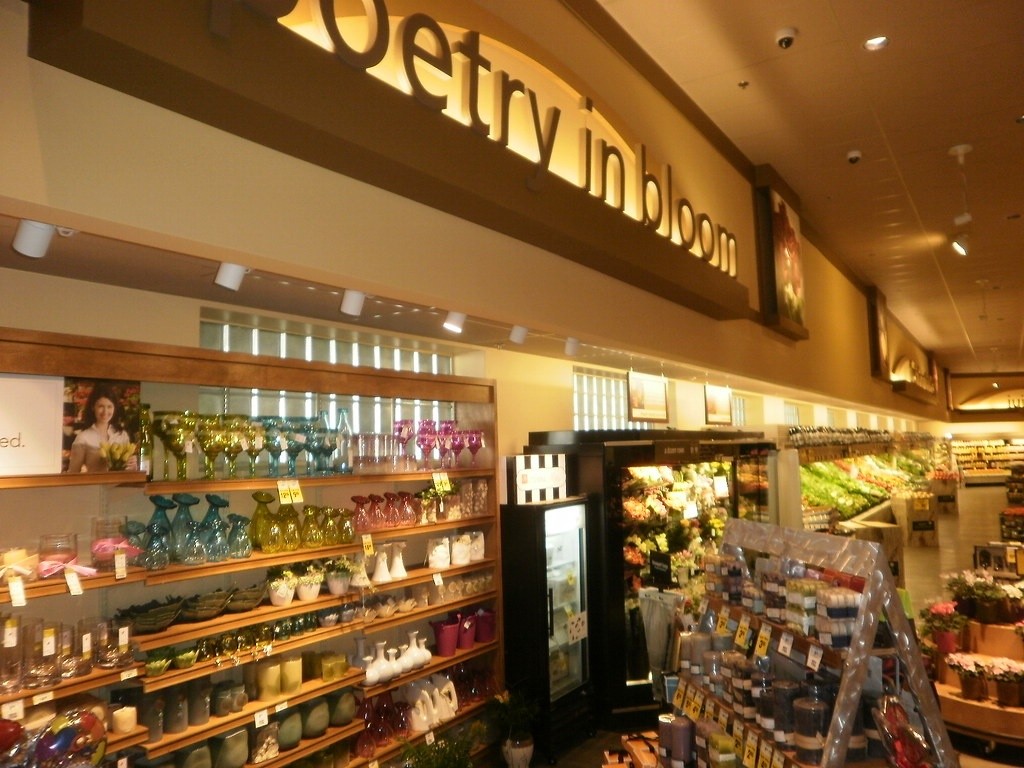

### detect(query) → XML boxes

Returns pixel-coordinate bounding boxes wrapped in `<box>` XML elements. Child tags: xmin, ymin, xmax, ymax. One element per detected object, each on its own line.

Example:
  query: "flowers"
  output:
<box><xmin>294</xmin><ymin>561</ymin><xmax>324</xmax><ymax>586</ymax></box>
<box><xmin>266</xmin><ymin>565</ymin><xmax>297</xmax><ymax>589</ymax></box>
<box><xmin>497</xmin><ymin>690</ymin><xmax>534</xmax><ymax>737</ymax></box>
<box><xmin>323</xmin><ymin>553</ymin><xmax>361</xmax><ymax>577</ymax></box>
<box><xmin>916</xmin><ymin>636</ymin><xmax>939</xmax><ymax>652</ymax></box>
<box><xmin>1004</xmin><ymin>581</ymin><xmax>1024</xmax><ymax>600</ymax></box>
<box><xmin>988</xmin><ymin>656</ymin><xmax>1024</xmax><ymax>682</ymax></box>
<box><xmin>919</xmin><ymin>597</ymin><xmax>967</xmax><ymax>636</ymax></box>
<box><xmin>937</xmin><ymin>567</ymin><xmax>1007</xmax><ymax>599</ymax></box>
<box><xmin>416</xmin><ymin>481</ymin><xmax>459</xmax><ymax>503</ymax></box>
<box><xmin>944</xmin><ymin>650</ymin><xmax>993</xmax><ymax>677</ymax></box>
<box><xmin>100</xmin><ymin>439</ymin><xmax>137</xmax><ymax>471</ymax></box>
<box><xmin>398</xmin><ymin>730</ymin><xmax>477</xmax><ymax>768</ymax></box>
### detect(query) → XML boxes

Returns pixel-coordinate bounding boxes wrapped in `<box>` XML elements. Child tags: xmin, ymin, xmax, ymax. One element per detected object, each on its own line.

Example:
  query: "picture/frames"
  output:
<box><xmin>703</xmin><ymin>384</ymin><xmax>733</xmax><ymax>425</ymax></box>
<box><xmin>627</xmin><ymin>368</ymin><xmax>670</xmax><ymax>423</ymax></box>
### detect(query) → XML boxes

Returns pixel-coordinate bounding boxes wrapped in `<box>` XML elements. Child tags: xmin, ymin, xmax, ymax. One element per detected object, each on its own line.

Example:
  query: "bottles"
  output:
<box><xmin>350</xmin><ymin>491</ymin><xmax>416</xmax><ymax>531</ymax></box>
<box><xmin>250</xmin><ymin>489</ymin><xmax>355</xmax><ymax>553</ymax></box>
<box><xmin>352</xmin><ymin>629</ymin><xmax>431</xmax><ymax>687</ymax></box>
<box><xmin>349</xmin><ymin>540</ymin><xmax>408</xmax><ymax>587</ymax></box>
<box><xmin>140</xmin><ymin>402</ymin><xmax>153</xmax><ymax>481</ymax></box>
<box><xmin>119</xmin><ymin>492</ymin><xmax>252</xmax><ymax>570</ymax></box>
<box><xmin>355</xmin><ymin>686</ymin><xmax>411</xmax><ymax>758</ymax></box>
<box><xmin>312</xmin><ymin>407</ymin><xmax>353</xmax><ymax>477</ymax></box>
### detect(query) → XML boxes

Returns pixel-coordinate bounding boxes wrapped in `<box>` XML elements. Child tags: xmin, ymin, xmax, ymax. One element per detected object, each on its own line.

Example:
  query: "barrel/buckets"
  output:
<box><xmin>428</xmin><ymin>607</ymin><xmax>496</xmax><ymax>658</ymax></box>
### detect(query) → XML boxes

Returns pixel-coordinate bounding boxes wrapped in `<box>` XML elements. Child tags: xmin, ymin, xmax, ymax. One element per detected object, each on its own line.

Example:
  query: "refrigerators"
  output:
<box><xmin>500</xmin><ymin>492</ymin><xmax>609</xmax><ymax>766</ymax></box>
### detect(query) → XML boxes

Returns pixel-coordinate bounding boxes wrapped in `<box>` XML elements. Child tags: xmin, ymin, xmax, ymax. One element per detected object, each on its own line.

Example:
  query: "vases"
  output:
<box><xmin>976</xmin><ymin>600</ymin><xmax>999</xmax><ymax>622</ymax></box>
<box><xmin>959</xmin><ymin>675</ymin><xmax>986</xmax><ymax>700</ymax></box>
<box><xmin>500</xmin><ymin>732</ymin><xmax>534</xmax><ymax>768</ymax></box>
<box><xmin>958</xmin><ymin>600</ymin><xmax>975</xmax><ymax>618</ymax></box>
<box><xmin>932</xmin><ymin>631</ymin><xmax>960</xmax><ymax>653</ymax></box>
<box><xmin>998</xmin><ymin>681</ymin><xmax>1023</xmax><ymax>706</ymax></box>
<box><xmin>0</xmin><ymin>406</ymin><xmax>494</xmax><ymax>768</ymax></box>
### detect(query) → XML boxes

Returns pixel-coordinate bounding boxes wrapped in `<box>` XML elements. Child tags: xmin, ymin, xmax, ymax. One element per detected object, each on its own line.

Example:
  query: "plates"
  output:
<box><xmin>112</xmin><ymin>578</ymin><xmax>269</xmax><ymax>633</ymax></box>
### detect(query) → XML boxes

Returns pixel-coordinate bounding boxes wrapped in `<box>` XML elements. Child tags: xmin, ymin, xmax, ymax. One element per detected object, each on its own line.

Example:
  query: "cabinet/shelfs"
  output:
<box><xmin>577</xmin><ymin>431</ymin><xmax>766</xmax><ymax>726</ymax></box>
<box><xmin>500</xmin><ymin>495</ymin><xmax>595</xmax><ymax>756</ymax></box>
<box><xmin>1005</xmin><ymin>482</ymin><xmax>1024</xmax><ymax>506</ymax></box>
<box><xmin>0</xmin><ymin>331</ymin><xmax>509</xmax><ymax>768</ymax></box>
<box><xmin>686</xmin><ymin>515</ymin><xmax>961</xmax><ymax>768</ymax></box>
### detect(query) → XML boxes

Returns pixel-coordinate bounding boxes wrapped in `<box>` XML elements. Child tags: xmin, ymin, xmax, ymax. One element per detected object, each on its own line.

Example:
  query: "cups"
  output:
<box><xmin>38</xmin><ymin>532</ymin><xmax>78</xmax><ymax>578</ymax></box>
<box><xmin>90</xmin><ymin>515</ymin><xmax>128</xmax><ymax>572</ymax></box>
<box><xmin>0</xmin><ymin>612</ymin><xmax>134</xmax><ymax>696</ymax></box>
<box><xmin>427</xmin><ymin>530</ymin><xmax>486</xmax><ymax>569</ymax></box>
<box><xmin>353</xmin><ymin>433</ymin><xmax>418</xmax><ymax>476</ymax></box>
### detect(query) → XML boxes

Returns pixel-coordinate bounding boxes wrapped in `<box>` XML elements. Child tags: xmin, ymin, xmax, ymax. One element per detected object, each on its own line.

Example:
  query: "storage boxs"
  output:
<box><xmin>837</xmin><ymin>521</ymin><xmax>905</xmax><ymax>587</ymax></box>
<box><xmin>890</xmin><ymin>493</ymin><xmax>939</xmax><ymax>546</ymax></box>
<box><xmin>999</xmin><ymin>508</ymin><xmax>1024</xmax><ymax>543</ymax></box>
<box><xmin>931</xmin><ymin>478</ymin><xmax>959</xmax><ymax>516</ymax></box>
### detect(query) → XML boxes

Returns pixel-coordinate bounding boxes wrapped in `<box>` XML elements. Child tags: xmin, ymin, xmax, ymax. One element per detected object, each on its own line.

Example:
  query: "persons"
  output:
<box><xmin>68</xmin><ymin>383</ymin><xmax>137</xmax><ymax>472</ymax></box>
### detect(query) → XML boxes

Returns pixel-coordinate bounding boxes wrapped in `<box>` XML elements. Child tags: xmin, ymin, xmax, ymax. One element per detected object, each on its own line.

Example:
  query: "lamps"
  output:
<box><xmin>563</xmin><ymin>337</ymin><xmax>579</xmax><ymax>355</ymax></box>
<box><xmin>214</xmin><ymin>262</ymin><xmax>245</xmax><ymax>288</ymax></box>
<box><xmin>13</xmin><ymin>220</ymin><xmax>55</xmax><ymax>257</ymax></box>
<box><xmin>951</xmin><ymin>232</ymin><xmax>974</xmax><ymax>258</ymax></box>
<box><xmin>444</xmin><ymin>311</ymin><xmax>466</xmax><ymax>334</ymax></box>
<box><xmin>338</xmin><ymin>289</ymin><xmax>363</xmax><ymax>316</ymax></box>
<box><xmin>509</xmin><ymin>325</ymin><xmax>528</xmax><ymax>345</ymax></box>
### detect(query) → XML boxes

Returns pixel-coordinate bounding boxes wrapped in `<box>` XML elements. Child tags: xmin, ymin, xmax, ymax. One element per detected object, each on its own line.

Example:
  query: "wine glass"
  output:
<box><xmin>153</xmin><ymin>411</ymin><xmax>339</xmax><ymax>482</ymax></box>
<box><xmin>393</xmin><ymin>419</ymin><xmax>484</xmax><ymax>468</ymax></box>
